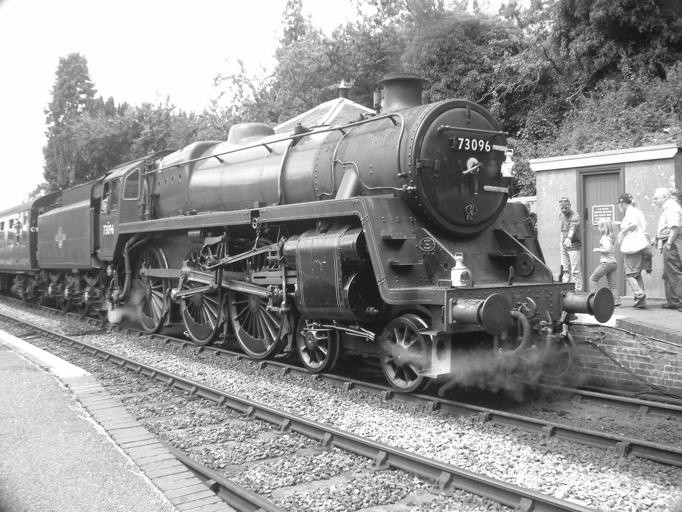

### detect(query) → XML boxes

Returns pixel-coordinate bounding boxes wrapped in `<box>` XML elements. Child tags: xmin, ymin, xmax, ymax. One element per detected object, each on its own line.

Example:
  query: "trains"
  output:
<box><xmin>0</xmin><ymin>73</ymin><xmax>617</xmax><ymax>397</ymax></box>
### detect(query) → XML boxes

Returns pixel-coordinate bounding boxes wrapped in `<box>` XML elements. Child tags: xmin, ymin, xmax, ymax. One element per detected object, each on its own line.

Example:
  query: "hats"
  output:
<box><xmin>613</xmin><ymin>193</ymin><xmax>632</xmax><ymax>206</ymax></box>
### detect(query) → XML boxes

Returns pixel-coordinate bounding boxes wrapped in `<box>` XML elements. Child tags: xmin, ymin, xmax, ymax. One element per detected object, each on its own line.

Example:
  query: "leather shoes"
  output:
<box><xmin>663</xmin><ymin>303</ymin><xmax>682</xmax><ymax>312</ymax></box>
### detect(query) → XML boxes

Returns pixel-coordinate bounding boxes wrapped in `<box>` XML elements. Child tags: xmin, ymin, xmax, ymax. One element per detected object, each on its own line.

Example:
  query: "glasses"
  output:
<box><xmin>558</xmin><ymin>198</ymin><xmax>571</xmax><ymax>204</ymax></box>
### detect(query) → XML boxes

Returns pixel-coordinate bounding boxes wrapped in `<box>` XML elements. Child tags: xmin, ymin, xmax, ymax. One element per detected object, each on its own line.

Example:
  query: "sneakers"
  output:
<box><xmin>631</xmin><ymin>292</ymin><xmax>647</xmax><ymax>309</ymax></box>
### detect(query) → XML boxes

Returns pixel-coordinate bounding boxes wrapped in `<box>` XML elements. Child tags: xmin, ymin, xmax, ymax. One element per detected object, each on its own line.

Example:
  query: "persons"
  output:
<box><xmin>588</xmin><ymin>218</ymin><xmax>623</xmax><ymax>307</ymax></box>
<box><xmin>614</xmin><ymin>193</ymin><xmax>648</xmax><ymax>309</ymax></box>
<box><xmin>653</xmin><ymin>186</ymin><xmax>681</xmax><ymax>310</ymax></box>
<box><xmin>530</xmin><ymin>212</ymin><xmax>539</xmax><ymax>232</ymax></box>
<box><xmin>557</xmin><ymin>197</ymin><xmax>583</xmax><ymax>292</ymax></box>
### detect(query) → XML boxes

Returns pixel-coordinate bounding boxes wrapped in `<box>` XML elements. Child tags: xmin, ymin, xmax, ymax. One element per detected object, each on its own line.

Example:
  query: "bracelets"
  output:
<box><xmin>666</xmin><ymin>241</ymin><xmax>671</xmax><ymax>246</ymax></box>
